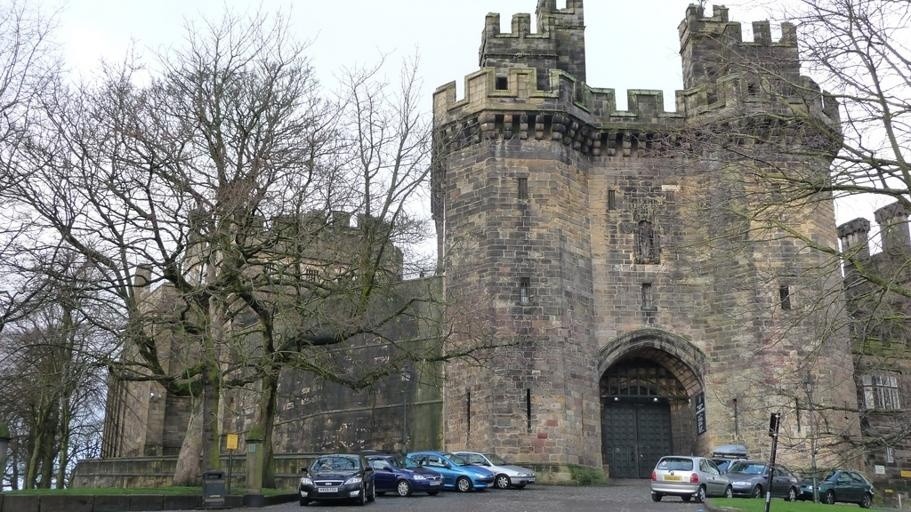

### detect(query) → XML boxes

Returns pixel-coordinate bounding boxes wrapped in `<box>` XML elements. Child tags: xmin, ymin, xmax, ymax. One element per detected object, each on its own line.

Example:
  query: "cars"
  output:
<box><xmin>722</xmin><ymin>460</ymin><xmax>800</xmax><ymax>501</ymax></box>
<box><xmin>710</xmin><ymin>457</ymin><xmax>749</xmax><ymax>474</ymax></box>
<box><xmin>650</xmin><ymin>455</ymin><xmax>735</xmax><ymax>503</ymax></box>
<box><xmin>300</xmin><ymin>453</ymin><xmax>376</xmax><ymax>506</ymax></box>
<box><xmin>452</xmin><ymin>451</ymin><xmax>536</xmax><ymax>489</ymax></box>
<box><xmin>800</xmin><ymin>469</ymin><xmax>876</xmax><ymax>508</ymax></box>
<box><xmin>406</xmin><ymin>450</ymin><xmax>496</xmax><ymax>492</ymax></box>
<box><xmin>365</xmin><ymin>452</ymin><xmax>445</xmax><ymax>497</ymax></box>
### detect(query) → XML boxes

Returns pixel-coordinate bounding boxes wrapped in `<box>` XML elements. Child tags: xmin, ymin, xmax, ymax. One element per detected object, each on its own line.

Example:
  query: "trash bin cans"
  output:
<box><xmin>202</xmin><ymin>471</ymin><xmax>225</xmax><ymax>509</ymax></box>
